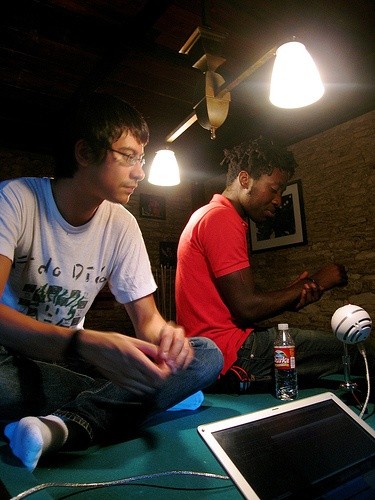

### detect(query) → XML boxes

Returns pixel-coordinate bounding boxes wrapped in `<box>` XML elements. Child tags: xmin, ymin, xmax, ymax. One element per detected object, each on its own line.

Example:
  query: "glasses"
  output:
<box><xmin>96</xmin><ymin>142</ymin><xmax>146</xmax><ymax>167</ymax></box>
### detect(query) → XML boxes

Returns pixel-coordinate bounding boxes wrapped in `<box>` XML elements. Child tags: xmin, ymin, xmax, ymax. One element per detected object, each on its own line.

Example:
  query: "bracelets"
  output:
<box><xmin>304</xmin><ymin>276</ymin><xmax>320</xmax><ymax>288</ymax></box>
<box><xmin>65</xmin><ymin>327</ymin><xmax>88</xmax><ymax>365</ymax></box>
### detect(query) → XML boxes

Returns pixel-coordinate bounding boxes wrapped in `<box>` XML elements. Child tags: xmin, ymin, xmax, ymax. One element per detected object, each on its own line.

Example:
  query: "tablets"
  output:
<box><xmin>198</xmin><ymin>391</ymin><xmax>375</xmax><ymax>500</ymax></box>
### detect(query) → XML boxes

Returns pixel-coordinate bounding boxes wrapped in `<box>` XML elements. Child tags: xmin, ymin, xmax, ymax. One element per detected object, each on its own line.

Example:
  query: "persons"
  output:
<box><xmin>175</xmin><ymin>137</ymin><xmax>375</xmax><ymax>406</ymax></box>
<box><xmin>0</xmin><ymin>91</ymin><xmax>225</xmax><ymax>473</ymax></box>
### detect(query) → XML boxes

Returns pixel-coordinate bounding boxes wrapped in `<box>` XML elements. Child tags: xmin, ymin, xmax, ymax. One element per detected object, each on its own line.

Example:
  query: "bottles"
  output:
<box><xmin>274</xmin><ymin>323</ymin><xmax>298</xmax><ymax>401</ymax></box>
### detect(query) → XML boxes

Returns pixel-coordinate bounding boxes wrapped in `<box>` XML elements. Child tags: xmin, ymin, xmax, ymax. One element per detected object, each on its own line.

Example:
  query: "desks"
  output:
<box><xmin>0</xmin><ymin>378</ymin><xmax>375</xmax><ymax>500</ymax></box>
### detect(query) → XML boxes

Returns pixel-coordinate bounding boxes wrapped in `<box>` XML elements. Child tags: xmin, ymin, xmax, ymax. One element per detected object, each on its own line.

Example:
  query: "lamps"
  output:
<box><xmin>148</xmin><ymin>150</ymin><xmax>180</xmax><ymax>186</ymax></box>
<box><xmin>269</xmin><ymin>42</ymin><xmax>324</xmax><ymax>109</ymax></box>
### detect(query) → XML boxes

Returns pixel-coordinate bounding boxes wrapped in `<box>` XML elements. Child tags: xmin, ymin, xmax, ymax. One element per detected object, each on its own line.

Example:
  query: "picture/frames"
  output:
<box><xmin>159</xmin><ymin>241</ymin><xmax>177</xmax><ymax>269</ymax></box>
<box><xmin>247</xmin><ymin>179</ymin><xmax>308</xmax><ymax>255</ymax></box>
<box><xmin>139</xmin><ymin>193</ymin><xmax>166</xmax><ymax>221</ymax></box>
